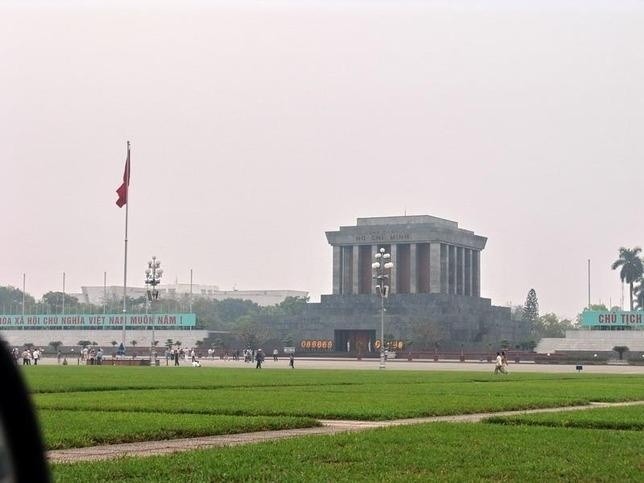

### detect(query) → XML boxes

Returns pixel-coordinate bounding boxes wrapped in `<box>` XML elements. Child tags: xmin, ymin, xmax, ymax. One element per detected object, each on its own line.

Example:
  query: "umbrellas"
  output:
<box><xmin>116</xmin><ymin>343</ymin><xmax>124</xmax><ymax>360</ymax></box>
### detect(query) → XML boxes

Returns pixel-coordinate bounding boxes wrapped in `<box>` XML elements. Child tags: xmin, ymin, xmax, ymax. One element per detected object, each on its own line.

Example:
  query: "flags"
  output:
<box><xmin>114</xmin><ymin>148</ymin><xmax>131</xmax><ymax>209</ymax></box>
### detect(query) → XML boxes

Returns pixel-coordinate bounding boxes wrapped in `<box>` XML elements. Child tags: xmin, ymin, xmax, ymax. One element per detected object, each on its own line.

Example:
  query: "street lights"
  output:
<box><xmin>372</xmin><ymin>247</ymin><xmax>393</xmax><ymax>369</ymax></box>
<box><xmin>145</xmin><ymin>255</ymin><xmax>163</xmax><ymax>368</ymax></box>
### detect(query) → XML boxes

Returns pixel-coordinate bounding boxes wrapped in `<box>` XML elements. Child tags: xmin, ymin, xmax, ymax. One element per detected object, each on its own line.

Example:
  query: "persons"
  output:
<box><xmin>9</xmin><ymin>345</ymin><xmax>296</xmax><ymax>370</ymax></box>
<box><xmin>493</xmin><ymin>352</ymin><xmax>510</xmax><ymax>376</ymax></box>
<box><xmin>384</xmin><ymin>348</ymin><xmax>388</xmax><ymax>360</ymax></box>
<box><xmin>500</xmin><ymin>351</ymin><xmax>508</xmax><ymax>369</ymax></box>
